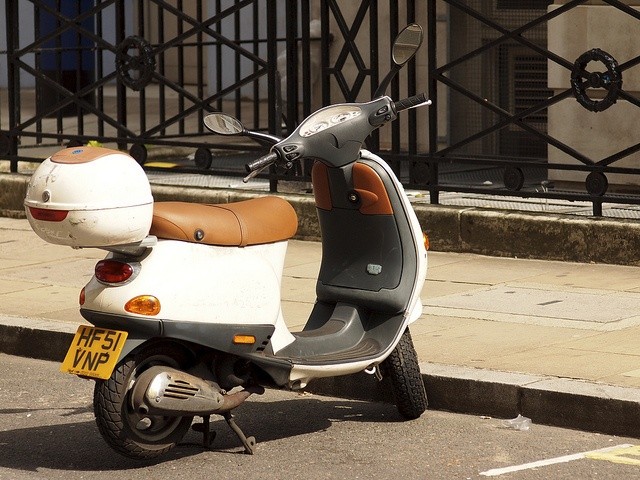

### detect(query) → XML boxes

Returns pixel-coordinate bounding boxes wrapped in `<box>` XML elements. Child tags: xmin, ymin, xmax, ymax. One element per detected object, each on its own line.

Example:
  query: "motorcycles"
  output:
<box><xmin>24</xmin><ymin>24</ymin><xmax>429</xmax><ymax>459</ymax></box>
